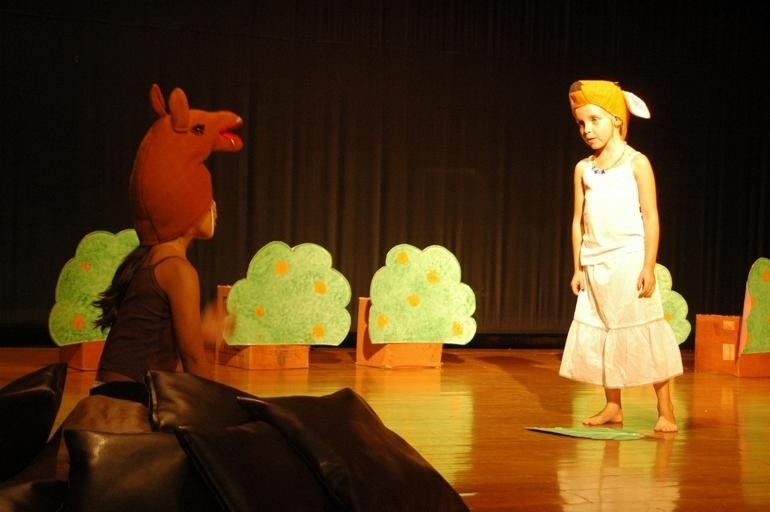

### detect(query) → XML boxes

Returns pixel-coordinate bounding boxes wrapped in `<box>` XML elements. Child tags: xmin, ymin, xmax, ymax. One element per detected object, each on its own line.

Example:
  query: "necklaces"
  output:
<box><xmin>591</xmin><ymin>146</ymin><xmax>628</xmax><ymax>175</ymax></box>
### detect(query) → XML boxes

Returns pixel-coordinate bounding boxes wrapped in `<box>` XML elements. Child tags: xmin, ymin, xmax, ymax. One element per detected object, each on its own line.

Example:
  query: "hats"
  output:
<box><xmin>570</xmin><ymin>79</ymin><xmax>651</xmax><ymax>141</ymax></box>
<box><xmin>126</xmin><ymin>86</ymin><xmax>242</xmax><ymax>246</ymax></box>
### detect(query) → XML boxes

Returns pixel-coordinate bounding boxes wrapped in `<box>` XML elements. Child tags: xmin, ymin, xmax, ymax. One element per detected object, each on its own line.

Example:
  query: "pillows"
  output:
<box><xmin>234</xmin><ymin>385</ymin><xmax>469</xmax><ymax>509</ymax></box>
<box><xmin>3</xmin><ymin>360</ymin><xmax>70</xmax><ymax>486</ymax></box>
<box><xmin>87</xmin><ymin>380</ymin><xmax>151</xmax><ymax>405</ymax></box>
<box><xmin>62</xmin><ymin>425</ymin><xmax>198</xmax><ymax>511</ymax></box>
<box><xmin>174</xmin><ymin>418</ymin><xmax>338</xmax><ymax>508</ymax></box>
<box><xmin>2</xmin><ymin>392</ymin><xmax>159</xmax><ymax>511</ymax></box>
<box><xmin>144</xmin><ymin>365</ymin><xmax>358</xmax><ymax>509</ymax></box>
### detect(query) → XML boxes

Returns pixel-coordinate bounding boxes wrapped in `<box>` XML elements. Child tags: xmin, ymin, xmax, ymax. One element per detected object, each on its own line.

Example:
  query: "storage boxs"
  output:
<box><xmin>215</xmin><ymin>284</ymin><xmax>444</xmax><ymax>371</ymax></box>
<box><xmin>694</xmin><ymin>313</ymin><xmax>769</xmax><ymax>380</ymax></box>
<box><xmin>61</xmin><ymin>342</ymin><xmax>105</xmax><ymax>371</ymax></box>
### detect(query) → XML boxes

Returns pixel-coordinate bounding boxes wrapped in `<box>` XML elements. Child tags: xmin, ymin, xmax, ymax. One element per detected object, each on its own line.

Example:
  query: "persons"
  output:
<box><xmin>592</xmin><ymin>428</ymin><xmax>676</xmax><ymax>512</ymax></box>
<box><xmin>555</xmin><ymin>81</ymin><xmax>681</xmax><ymax>435</ymax></box>
<box><xmin>0</xmin><ymin>82</ymin><xmax>249</xmax><ymax>510</ymax></box>
